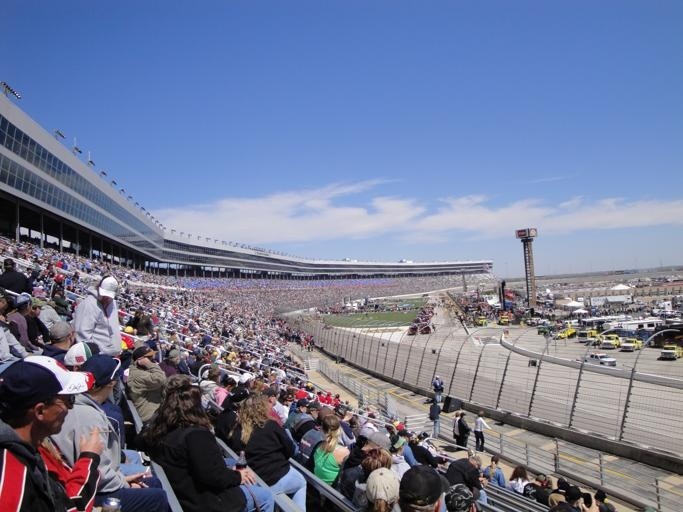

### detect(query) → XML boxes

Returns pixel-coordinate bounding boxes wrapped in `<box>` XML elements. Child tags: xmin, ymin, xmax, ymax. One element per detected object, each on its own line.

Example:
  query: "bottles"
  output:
<box><xmin>236</xmin><ymin>450</ymin><xmax>247</xmax><ymax>470</ymax></box>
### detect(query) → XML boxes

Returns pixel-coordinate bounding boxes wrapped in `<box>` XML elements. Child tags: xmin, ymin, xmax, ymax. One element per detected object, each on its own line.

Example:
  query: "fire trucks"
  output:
<box><xmin>454</xmin><ymin>294</ymin><xmax>683</xmax><ymax>360</ymax></box>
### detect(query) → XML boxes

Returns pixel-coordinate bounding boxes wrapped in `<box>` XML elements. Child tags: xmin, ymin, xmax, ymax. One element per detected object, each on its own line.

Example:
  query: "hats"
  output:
<box><xmin>471</xmin><ymin>452</ymin><xmax>608</xmax><ymax>503</ymax></box>
<box><xmin>230</xmin><ymin>372</ymin><xmax>450</xmax><ymax>506</ymax></box>
<box><xmin>0</xmin><ymin>258</ymin><xmax>180</xmax><ymax>408</ymax></box>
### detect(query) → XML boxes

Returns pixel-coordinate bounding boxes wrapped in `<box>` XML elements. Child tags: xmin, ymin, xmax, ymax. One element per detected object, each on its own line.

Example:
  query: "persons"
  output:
<box><xmin>314</xmin><ymin>273</ymin><xmax>495</xmax><ymax>332</ymax></box>
<box><xmin>539</xmin><ymin>304</ymin><xmax>683</xmax><ymax>346</ymax></box>
<box><xmin>483</xmin><ymin>455</ymin><xmax>616</xmax><ymax>512</ymax></box>
<box><xmin>432</xmin><ymin>377</ymin><xmax>444</xmax><ymax>402</ymax></box>
<box><xmin>1</xmin><ymin>235</ymin><xmax>315</xmax><ymax>506</ymax></box>
<box><xmin>313</xmin><ymin>387</ymin><xmax>492</xmax><ymax>512</ymax></box>
